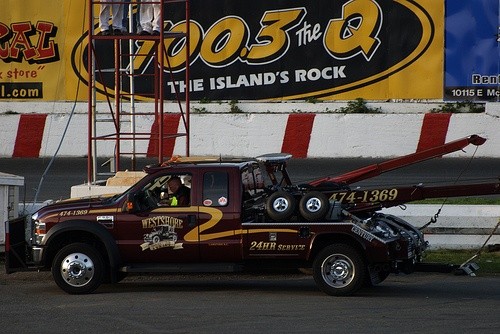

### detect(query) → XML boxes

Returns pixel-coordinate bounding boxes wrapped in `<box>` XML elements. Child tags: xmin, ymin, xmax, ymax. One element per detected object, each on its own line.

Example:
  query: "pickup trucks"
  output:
<box><xmin>30</xmin><ymin>133</ymin><xmax>499</xmax><ymax>298</ymax></box>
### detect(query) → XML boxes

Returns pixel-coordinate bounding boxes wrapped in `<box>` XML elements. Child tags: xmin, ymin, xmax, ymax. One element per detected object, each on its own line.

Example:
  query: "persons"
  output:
<box><xmin>162</xmin><ymin>174</ymin><xmax>192</xmax><ymax>207</ymax></box>
<box><xmin>99</xmin><ymin>0</ymin><xmax>127</xmax><ymax>36</ymax></box>
<box><xmin>139</xmin><ymin>0</ymin><xmax>163</xmax><ymax>37</ymax></box>
